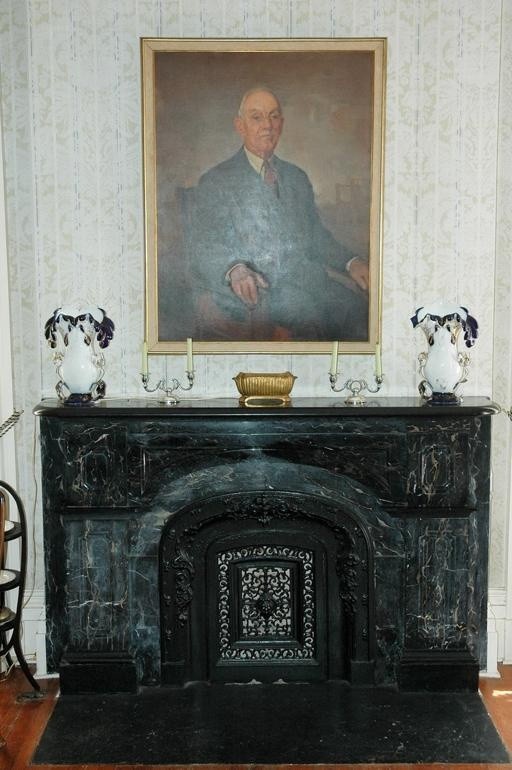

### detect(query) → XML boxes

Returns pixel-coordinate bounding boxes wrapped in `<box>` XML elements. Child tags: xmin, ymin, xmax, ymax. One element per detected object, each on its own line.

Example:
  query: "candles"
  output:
<box><xmin>186</xmin><ymin>338</ymin><xmax>193</xmax><ymax>374</ymax></box>
<box><xmin>375</xmin><ymin>344</ymin><xmax>382</xmax><ymax>378</ymax></box>
<box><xmin>142</xmin><ymin>343</ymin><xmax>148</xmax><ymax>375</ymax></box>
<box><xmin>330</xmin><ymin>340</ymin><xmax>338</xmax><ymax>376</ymax></box>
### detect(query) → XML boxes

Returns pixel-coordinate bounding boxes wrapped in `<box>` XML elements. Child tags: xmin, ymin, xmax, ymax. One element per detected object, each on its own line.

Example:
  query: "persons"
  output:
<box><xmin>185</xmin><ymin>84</ymin><xmax>368</xmax><ymax>341</ymax></box>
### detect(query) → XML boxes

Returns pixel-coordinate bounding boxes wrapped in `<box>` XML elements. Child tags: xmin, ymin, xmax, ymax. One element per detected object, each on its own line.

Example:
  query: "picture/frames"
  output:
<box><xmin>139</xmin><ymin>37</ymin><xmax>386</xmax><ymax>357</ymax></box>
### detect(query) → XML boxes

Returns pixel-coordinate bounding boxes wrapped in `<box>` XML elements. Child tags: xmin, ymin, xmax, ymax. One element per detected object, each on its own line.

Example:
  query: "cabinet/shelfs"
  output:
<box><xmin>0</xmin><ymin>480</ymin><xmax>41</xmax><ymax>693</ymax></box>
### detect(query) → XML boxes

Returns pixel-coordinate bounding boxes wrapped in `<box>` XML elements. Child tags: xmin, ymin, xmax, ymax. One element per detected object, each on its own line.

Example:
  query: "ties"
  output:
<box><xmin>263</xmin><ymin>160</ymin><xmax>280</xmax><ymax>199</ymax></box>
